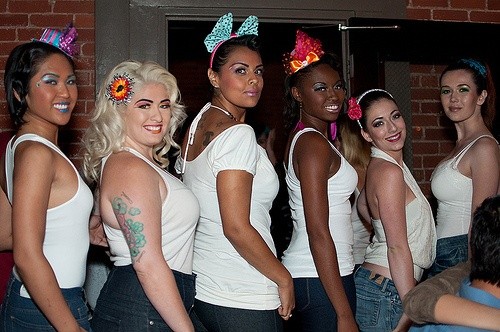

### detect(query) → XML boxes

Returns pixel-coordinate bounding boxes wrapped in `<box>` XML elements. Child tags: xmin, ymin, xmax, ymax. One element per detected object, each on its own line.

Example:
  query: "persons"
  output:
<box><xmin>175</xmin><ymin>12</ymin><xmax>295</xmax><ymax>332</ymax></box>
<box><xmin>334</xmin><ymin>131</ymin><xmax>374</xmax><ymax>274</ymax></box>
<box><xmin>0</xmin><ymin>27</ymin><xmax>108</xmax><ymax>332</ymax></box>
<box><xmin>281</xmin><ymin>30</ymin><xmax>360</xmax><ymax>332</ymax></box>
<box><xmin>347</xmin><ymin>89</ymin><xmax>437</xmax><ymax>332</ymax></box>
<box><xmin>401</xmin><ymin>195</ymin><xmax>500</xmax><ymax>332</ymax></box>
<box><xmin>430</xmin><ymin>57</ymin><xmax>500</xmax><ymax>276</ymax></box>
<box><xmin>82</xmin><ymin>61</ymin><xmax>208</xmax><ymax>332</ymax></box>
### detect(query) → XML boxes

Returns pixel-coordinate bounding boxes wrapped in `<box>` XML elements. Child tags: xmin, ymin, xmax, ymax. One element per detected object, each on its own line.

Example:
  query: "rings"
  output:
<box><xmin>288</xmin><ymin>313</ymin><xmax>292</xmax><ymax>318</ymax></box>
